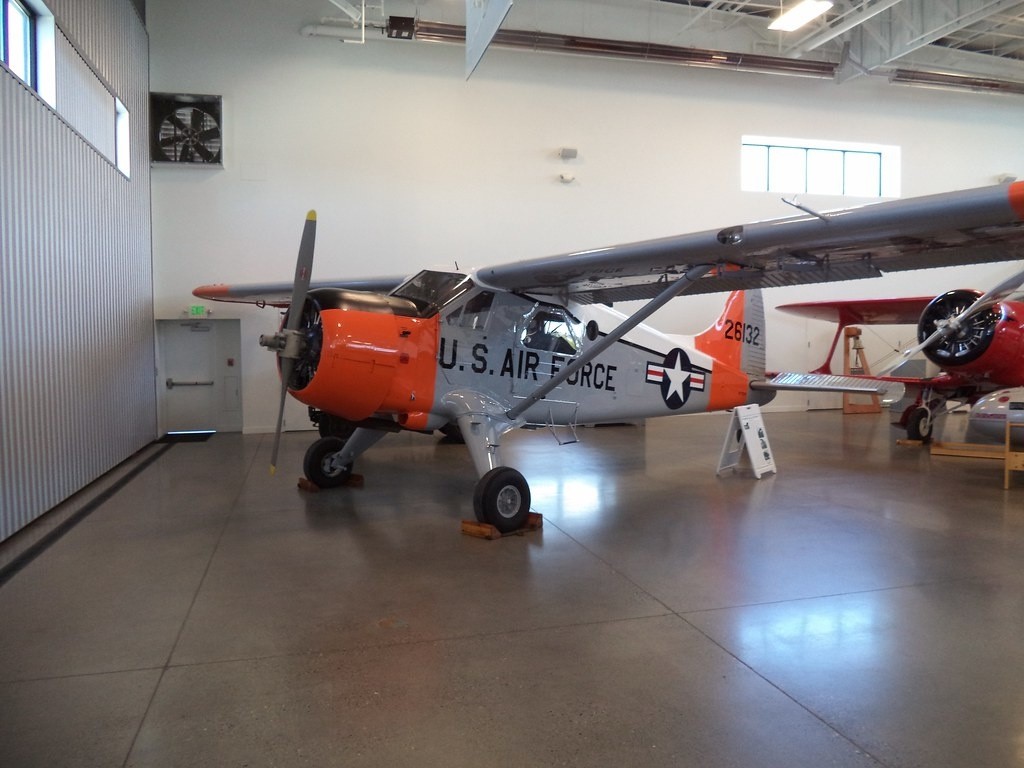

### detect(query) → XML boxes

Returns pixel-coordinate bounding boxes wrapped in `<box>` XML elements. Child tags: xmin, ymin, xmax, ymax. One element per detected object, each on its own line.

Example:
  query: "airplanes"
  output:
<box><xmin>773</xmin><ymin>287</ymin><xmax>1024</xmax><ymax>444</ymax></box>
<box><xmin>969</xmin><ymin>386</ymin><xmax>1024</xmax><ymax>448</ymax></box>
<box><xmin>192</xmin><ymin>179</ymin><xmax>1024</xmax><ymax>535</ymax></box>
